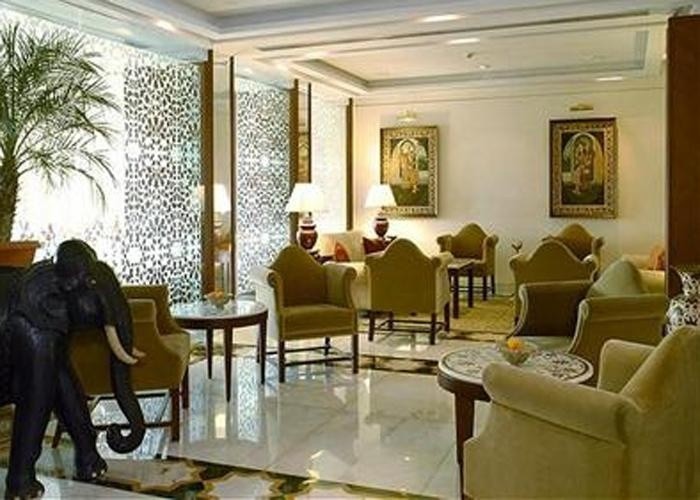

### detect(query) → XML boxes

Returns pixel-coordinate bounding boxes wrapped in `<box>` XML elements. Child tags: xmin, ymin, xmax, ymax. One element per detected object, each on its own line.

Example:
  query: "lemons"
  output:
<box><xmin>507</xmin><ymin>336</ymin><xmax>523</xmax><ymax>350</ymax></box>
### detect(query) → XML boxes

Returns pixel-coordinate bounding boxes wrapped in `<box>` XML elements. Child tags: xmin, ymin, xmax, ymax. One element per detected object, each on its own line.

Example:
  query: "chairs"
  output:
<box><xmin>500</xmin><ymin>267</ymin><xmax>671</xmax><ymax>375</ymax></box>
<box><xmin>616</xmin><ymin>244</ymin><xmax>667</xmax><ymax>293</ymax></box>
<box><xmin>505</xmin><ymin>237</ymin><xmax>600</xmax><ymax>327</ymax></box>
<box><xmin>461</xmin><ymin>322</ymin><xmax>698</xmax><ymax>498</ymax></box>
<box><xmin>537</xmin><ymin>221</ymin><xmax>608</xmax><ymax>284</ymax></box>
<box><xmin>435</xmin><ymin>222</ymin><xmax>500</xmax><ymax>302</ymax></box>
<box><xmin>247</xmin><ymin>243</ymin><xmax>362</xmax><ymax>385</ymax></box>
<box><xmin>363</xmin><ymin>236</ymin><xmax>452</xmax><ymax>345</ymax></box>
<box><xmin>44</xmin><ymin>281</ymin><xmax>195</xmax><ymax>448</ymax></box>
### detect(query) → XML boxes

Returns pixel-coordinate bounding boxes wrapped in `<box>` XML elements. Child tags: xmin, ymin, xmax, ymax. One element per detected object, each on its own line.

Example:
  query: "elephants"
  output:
<box><xmin>1</xmin><ymin>235</ymin><xmax>152</xmax><ymax>498</ymax></box>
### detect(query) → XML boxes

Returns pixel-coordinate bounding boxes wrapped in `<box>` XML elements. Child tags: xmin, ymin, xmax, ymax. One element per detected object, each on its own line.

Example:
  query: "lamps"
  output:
<box><xmin>283</xmin><ymin>182</ymin><xmax>327</xmax><ymax>248</ymax></box>
<box><xmin>364</xmin><ymin>183</ymin><xmax>398</xmax><ymax>240</ymax></box>
<box><xmin>398</xmin><ymin>109</ymin><xmax>418</xmax><ymax>124</ymax></box>
<box><xmin>568</xmin><ymin>98</ymin><xmax>595</xmax><ymax>112</ymax></box>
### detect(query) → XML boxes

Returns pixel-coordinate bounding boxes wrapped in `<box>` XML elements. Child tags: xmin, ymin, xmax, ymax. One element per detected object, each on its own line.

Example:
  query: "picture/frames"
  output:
<box><xmin>549</xmin><ymin>117</ymin><xmax>621</xmax><ymax>220</ymax></box>
<box><xmin>379</xmin><ymin>127</ymin><xmax>440</xmax><ymax>218</ymax></box>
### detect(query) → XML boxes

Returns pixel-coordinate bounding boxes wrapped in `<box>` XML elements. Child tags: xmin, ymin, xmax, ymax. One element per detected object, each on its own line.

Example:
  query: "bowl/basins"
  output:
<box><xmin>204</xmin><ymin>292</ymin><xmax>233</xmax><ymax>307</ymax></box>
<box><xmin>500</xmin><ymin>344</ymin><xmax>532</xmax><ymax>367</ymax></box>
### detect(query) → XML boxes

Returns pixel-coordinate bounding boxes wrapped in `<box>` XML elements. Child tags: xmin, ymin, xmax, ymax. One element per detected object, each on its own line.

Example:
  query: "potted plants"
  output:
<box><xmin>0</xmin><ymin>15</ymin><xmax>122</xmax><ymax>269</ymax></box>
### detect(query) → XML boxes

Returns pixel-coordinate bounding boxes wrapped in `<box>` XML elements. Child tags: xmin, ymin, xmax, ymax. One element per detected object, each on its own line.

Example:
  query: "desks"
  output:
<box><xmin>163</xmin><ymin>297</ymin><xmax>271</xmax><ymax>405</ymax></box>
<box><xmin>444</xmin><ymin>257</ymin><xmax>476</xmax><ymax>320</ymax></box>
<box><xmin>434</xmin><ymin>341</ymin><xmax>598</xmax><ymax>495</ymax></box>
